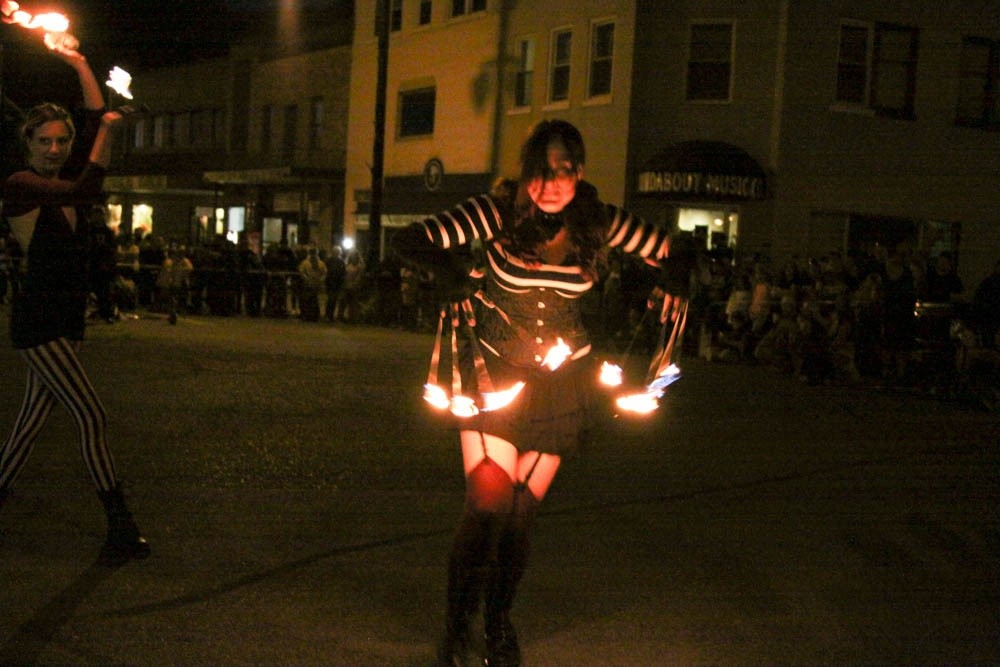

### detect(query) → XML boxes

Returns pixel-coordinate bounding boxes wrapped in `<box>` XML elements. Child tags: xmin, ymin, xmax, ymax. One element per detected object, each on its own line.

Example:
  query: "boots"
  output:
<box><xmin>436</xmin><ymin>533</ymin><xmax>494</xmax><ymax>667</ymax></box>
<box><xmin>96</xmin><ymin>478</ymin><xmax>151</xmax><ymax>555</ymax></box>
<box><xmin>484</xmin><ymin>526</ymin><xmax>531</xmax><ymax>667</ymax></box>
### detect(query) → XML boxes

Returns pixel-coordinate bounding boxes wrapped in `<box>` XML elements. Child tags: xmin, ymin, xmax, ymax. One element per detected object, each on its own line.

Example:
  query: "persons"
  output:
<box><xmin>596</xmin><ymin>228</ymin><xmax>1000</xmax><ymax>411</ymax></box>
<box><xmin>390</xmin><ymin>120</ymin><xmax>699</xmax><ymax>667</ymax></box>
<box><xmin>90</xmin><ymin>206</ymin><xmax>488</xmax><ymax>326</ymax></box>
<box><xmin>0</xmin><ymin>37</ymin><xmax>154</xmax><ymax>559</ymax></box>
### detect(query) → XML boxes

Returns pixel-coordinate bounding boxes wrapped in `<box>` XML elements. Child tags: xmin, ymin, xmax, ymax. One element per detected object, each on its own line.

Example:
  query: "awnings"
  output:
<box><xmin>634</xmin><ymin>136</ymin><xmax>766</xmax><ymax>199</ymax></box>
